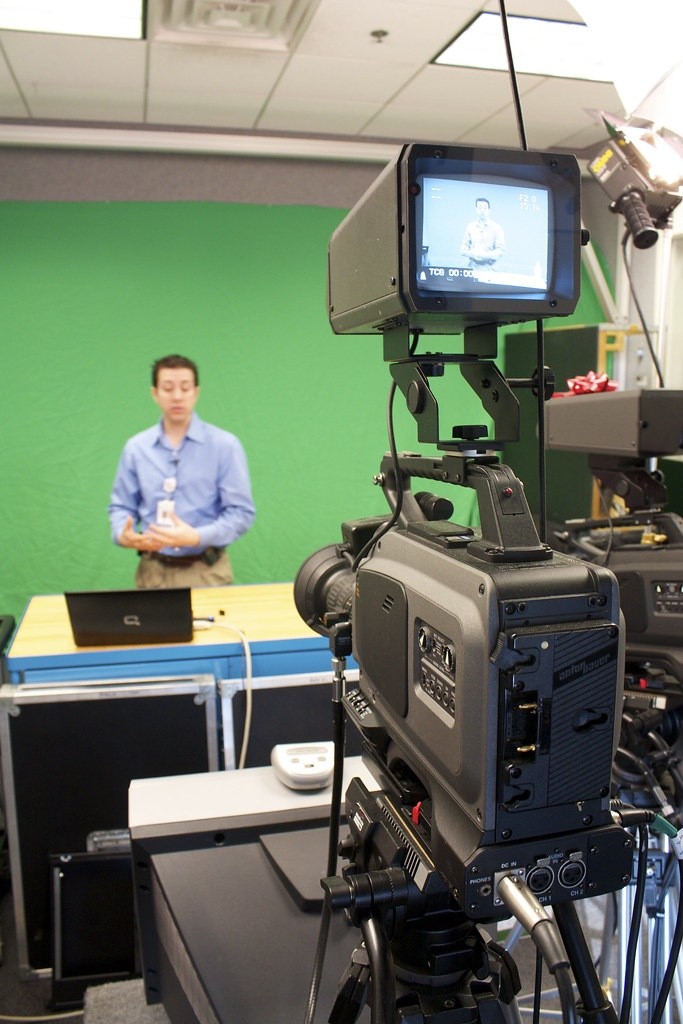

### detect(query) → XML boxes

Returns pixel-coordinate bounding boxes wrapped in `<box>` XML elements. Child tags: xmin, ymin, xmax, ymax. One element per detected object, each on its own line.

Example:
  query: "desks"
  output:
<box><xmin>2</xmin><ymin>583</ymin><xmax>360</xmax><ymax>686</ymax></box>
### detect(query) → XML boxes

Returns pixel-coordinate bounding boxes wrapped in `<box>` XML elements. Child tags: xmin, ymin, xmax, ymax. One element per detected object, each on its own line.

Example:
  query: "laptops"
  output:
<box><xmin>64</xmin><ymin>587</ymin><xmax>194</xmax><ymax>647</ymax></box>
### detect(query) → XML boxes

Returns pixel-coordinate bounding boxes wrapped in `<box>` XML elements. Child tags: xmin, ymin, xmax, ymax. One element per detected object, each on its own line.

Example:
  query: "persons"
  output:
<box><xmin>458</xmin><ymin>196</ymin><xmax>505</xmax><ymax>273</ymax></box>
<box><xmin>106</xmin><ymin>353</ymin><xmax>255</xmax><ymax>594</ymax></box>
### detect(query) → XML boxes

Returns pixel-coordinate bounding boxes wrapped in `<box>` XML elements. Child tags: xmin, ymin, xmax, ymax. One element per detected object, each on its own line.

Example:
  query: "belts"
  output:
<box><xmin>469</xmin><ymin>258</ymin><xmax>498</xmax><ymax>266</ymax></box>
<box><xmin>139</xmin><ymin>547</ymin><xmax>223</xmax><ymax>569</ymax></box>
